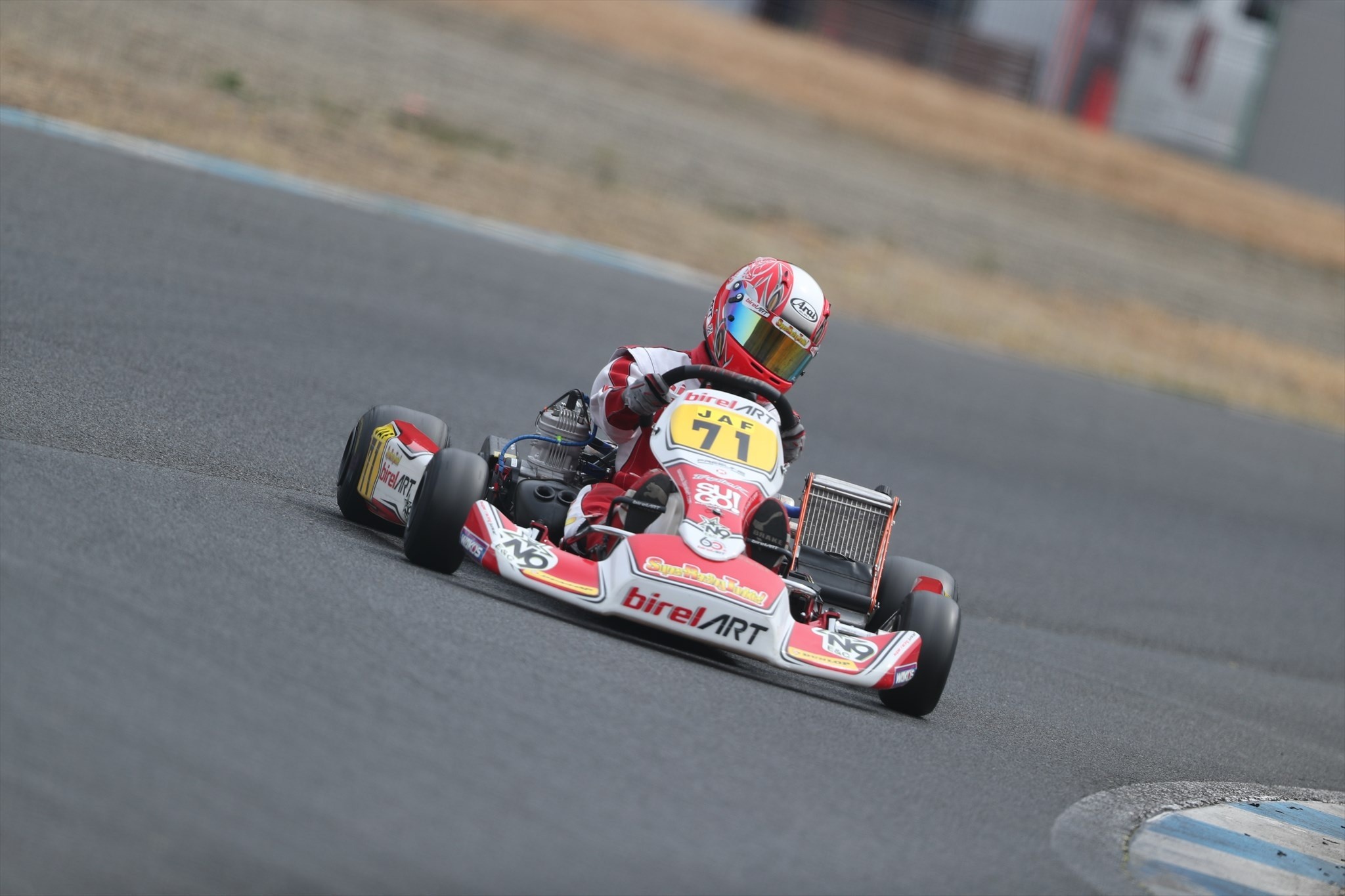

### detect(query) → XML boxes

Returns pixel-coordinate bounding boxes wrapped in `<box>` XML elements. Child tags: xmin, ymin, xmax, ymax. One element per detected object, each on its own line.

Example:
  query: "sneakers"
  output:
<box><xmin>744</xmin><ymin>496</ymin><xmax>791</xmax><ymax>574</ymax></box>
<box><xmin>586</xmin><ymin>468</ymin><xmax>672</xmax><ymax>562</ymax></box>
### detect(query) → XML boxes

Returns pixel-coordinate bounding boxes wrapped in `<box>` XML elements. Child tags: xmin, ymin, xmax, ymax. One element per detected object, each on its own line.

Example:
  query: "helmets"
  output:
<box><xmin>703</xmin><ymin>257</ymin><xmax>831</xmax><ymax>407</ymax></box>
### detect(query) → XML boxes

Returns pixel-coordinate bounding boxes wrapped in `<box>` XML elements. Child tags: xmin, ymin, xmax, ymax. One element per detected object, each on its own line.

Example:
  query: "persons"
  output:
<box><xmin>564</xmin><ymin>256</ymin><xmax>832</xmax><ymax>571</ymax></box>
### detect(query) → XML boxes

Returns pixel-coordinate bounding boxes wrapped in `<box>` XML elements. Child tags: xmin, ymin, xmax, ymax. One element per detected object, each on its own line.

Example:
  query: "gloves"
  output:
<box><xmin>622</xmin><ymin>373</ymin><xmax>671</xmax><ymax>417</ymax></box>
<box><xmin>779</xmin><ymin>423</ymin><xmax>806</xmax><ymax>464</ymax></box>
